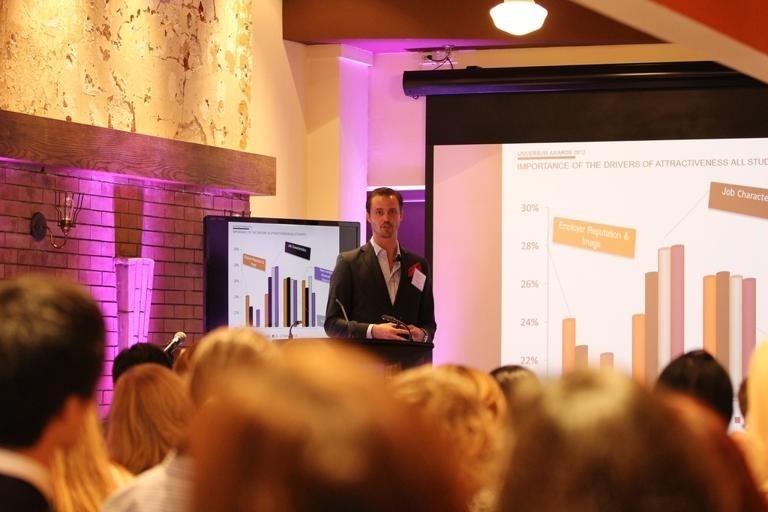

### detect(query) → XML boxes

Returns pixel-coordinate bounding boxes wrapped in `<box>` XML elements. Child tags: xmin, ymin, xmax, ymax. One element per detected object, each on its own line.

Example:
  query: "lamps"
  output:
<box><xmin>488</xmin><ymin>1</ymin><xmax>548</xmax><ymax>37</ymax></box>
<box><xmin>32</xmin><ymin>188</ymin><xmax>84</xmax><ymax>250</ymax></box>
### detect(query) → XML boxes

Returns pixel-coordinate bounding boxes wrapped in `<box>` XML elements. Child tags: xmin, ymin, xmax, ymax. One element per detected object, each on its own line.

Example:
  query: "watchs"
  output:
<box><xmin>420</xmin><ymin>325</ymin><xmax>428</xmax><ymax>343</ymax></box>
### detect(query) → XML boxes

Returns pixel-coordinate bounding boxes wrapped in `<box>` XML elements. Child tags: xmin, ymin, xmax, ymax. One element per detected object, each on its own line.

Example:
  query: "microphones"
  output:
<box><xmin>289</xmin><ymin>321</ymin><xmax>302</xmax><ymax>341</ymax></box>
<box><xmin>163</xmin><ymin>331</ymin><xmax>187</xmax><ymax>354</ymax></box>
<box><xmin>381</xmin><ymin>315</ymin><xmax>414</xmax><ymax>342</ymax></box>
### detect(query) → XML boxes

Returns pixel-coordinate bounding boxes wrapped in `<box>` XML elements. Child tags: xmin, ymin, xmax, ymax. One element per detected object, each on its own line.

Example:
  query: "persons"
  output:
<box><xmin>0</xmin><ymin>268</ymin><xmax>110</xmax><ymax>511</ymax></box>
<box><xmin>322</xmin><ymin>186</ymin><xmax>436</xmax><ymax>369</ymax></box>
<box><xmin>46</xmin><ymin>407</ymin><xmax>139</xmax><ymax>512</ymax></box>
<box><xmin>103</xmin><ymin>323</ymin><xmax>767</xmax><ymax>512</ymax></box>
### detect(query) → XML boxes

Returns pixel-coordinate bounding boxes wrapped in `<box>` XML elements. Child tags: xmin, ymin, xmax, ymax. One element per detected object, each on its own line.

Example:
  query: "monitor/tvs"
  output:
<box><xmin>202</xmin><ymin>215</ymin><xmax>361</xmax><ymax>341</ymax></box>
